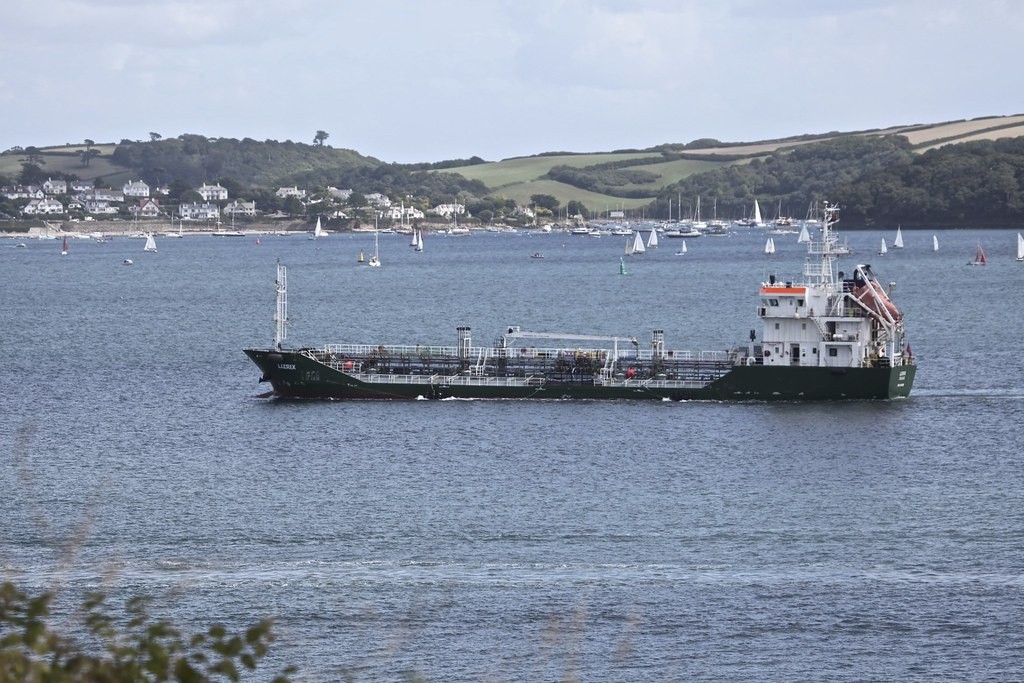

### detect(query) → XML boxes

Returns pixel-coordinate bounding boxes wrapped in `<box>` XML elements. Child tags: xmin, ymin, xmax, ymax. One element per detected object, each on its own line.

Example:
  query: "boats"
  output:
<box><xmin>540</xmin><ymin>224</ymin><xmax>551</xmax><ymax>234</ymax></box>
<box><xmin>240</xmin><ymin>197</ymin><xmax>919</xmax><ymax>402</ymax></box>
<box><xmin>279</xmin><ymin>231</ymin><xmax>291</xmax><ymax>235</ymax></box>
<box><xmin>382</xmin><ymin>228</ymin><xmax>394</xmax><ymax>234</ymax></box>
<box><xmin>353</xmin><ymin>226</ymin><xmax>381</xmax><ymax>232</ymax></box>
<box><xmin>16</xmin><ymin>241</ymin><xmax>24</xmax><ymax>248</ymax></box>
<box><xmin>75</xmin><ymin>234</ymin><xmax>89</xmax><ymax>238</ymax></box>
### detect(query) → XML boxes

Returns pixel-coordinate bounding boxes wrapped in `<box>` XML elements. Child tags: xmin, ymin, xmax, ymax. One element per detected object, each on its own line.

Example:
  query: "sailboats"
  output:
<box><xmin>39</xmin><ymin>221</ymin><xmax>56</xmax><ymax>240</ymax></box>
<box><xmin>1016</xmin><ymin>232</ymin><xmax>1024</xmax><ymax>260</ymax></box>
<box><xmin>763</xmin><ymin>238</ymin><xmax>775</xmax><ymax>255</ymax></box>
<box><xmin>967</xmin><ymin>237</ymin><xmax>986</xmax><ymax>266</ymax></box>
<box><xmin>877</xmin><ymin>237</ymin><xmax>887</xmax><ymax>256</ymax></box>
<box><xmin>646</xmin><ymin>227</ymin><xmax>658</xmax><ymax>247</ymax></box>
<box><xmin>368</xmin><ymin>214</ymin><xmax>381</xmax><ymax>267</ymax></box>
<box><xmin>933</xmin><ymin>234</ymin><xmax>939</xmax><ymax>251</ymax></box>
<box><xmin>798</xmin><ymin>223</ymin><xmax>811</xmax><ymax>244</ymax></box>
<box><xmin>889</xmin><ymin>223</ymin><xmax>903</xmax><ymax>248</ymax></box>
<box><xmin>410</xmin><ymin>226</ymin><xmax>417</xmax><ymax>247</ymax></box>
<box><xmin>178</xmin><ymin>219</ymin><xmax>183</xmax><ymax>238</ymax></box>
<box><xmin>211</xmin><ymin>204</ymin><xmax>247</xmax><ymax>237</ymax></box>
<box><xmin>308</xmin><ymin>215</ymin><xmax>320</xmax><ymax>242</ymax></box>
<box><xmin>62</xmin><ymin>233</ymin><xmax>68</xmax><ymax>254</ymax></box>
<box><xmin>144</xmin><ymin>231</ymin><xmax>158</xmax><ymax>252</ymax></box>
<box><xmin>320</xmin><ymin>232</ymin><xmax>328</xmax><ymax>236</ymax></box>
<box><xmin>130</xmin><ymin>205</ymin><xmax>148</xmax><ymax>239</ymax></box>
<box><xmin>483</xmin><ymin>193</ymin><xmax>823</xmax><ymax>236</ymax></box>
<box><xmin>415</xmin><ymin>228</ymin><xmax>424</xmax><ymax>253</ymax></box>
<box><xmin>96</xmin><ymin>240</ymin><xmax>107</xmax><ymax>243</ymax></box>
<box><xmin>625</xmin><ymin>230</ymin><xmax>645</xmax><ymax>256</ymax></box>
<box><xmin>675</xmin><ymin>241</ymin><xmax>687</xmax><ymax>257</ymax></box>
<box><xmin>436</xmin><ymin>198</ymin><xmax>470</xmax><ymax>235</ymax></box>
<box><xmin>397</xmin><ymin>200</ymin><xmax>413</xmax><ymax>235</ymax></box>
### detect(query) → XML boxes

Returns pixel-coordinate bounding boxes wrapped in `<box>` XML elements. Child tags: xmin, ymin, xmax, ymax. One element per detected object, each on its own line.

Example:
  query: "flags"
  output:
<box><xmin>906</xmin><ymin>343</ymin><xmax>912</xmax><ymax>356</ymax></box>
